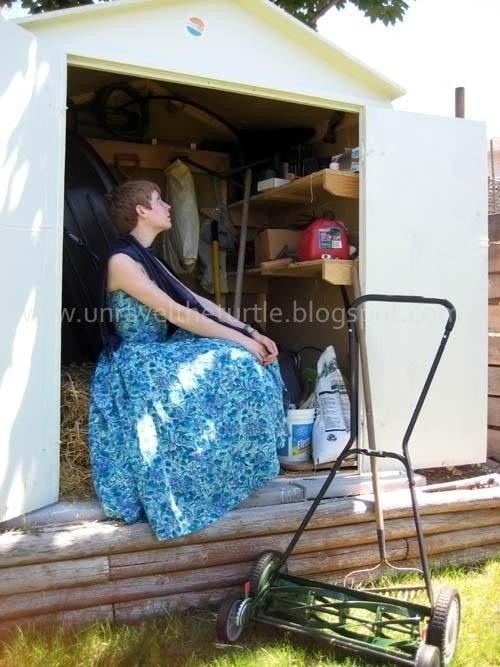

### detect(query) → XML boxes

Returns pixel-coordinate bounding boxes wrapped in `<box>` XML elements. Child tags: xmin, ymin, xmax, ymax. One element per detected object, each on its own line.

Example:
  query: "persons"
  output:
<box><xmin>87</xmin><ymin>180</ymin><xmax>291</xmax><ymax>541</ymax></box>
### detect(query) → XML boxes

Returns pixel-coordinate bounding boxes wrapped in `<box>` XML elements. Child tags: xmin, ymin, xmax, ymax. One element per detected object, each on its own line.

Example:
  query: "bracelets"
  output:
<box><xmin>243</xmin><ymin>324</ymin><xmax>249</xmax><ymax>332</ymax></box>
<box><xmin>249</xmin><ymin>329</ymin><xmax>257</xmax><ymax>336</ymax></box>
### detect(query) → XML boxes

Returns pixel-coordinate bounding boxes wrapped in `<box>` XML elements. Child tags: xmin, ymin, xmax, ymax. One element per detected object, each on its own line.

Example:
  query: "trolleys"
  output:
<box><xmin>216</xmin><ymin>291</ymin><xmax>461</xmax><ymax>667</ymax></box>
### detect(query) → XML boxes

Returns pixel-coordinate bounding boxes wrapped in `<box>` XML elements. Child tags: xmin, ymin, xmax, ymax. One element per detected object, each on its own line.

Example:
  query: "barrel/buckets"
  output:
<box><xmin>275</xmin><ymin>408</ymin><xmax>316</xmax><ymax>462</ymax></box>
